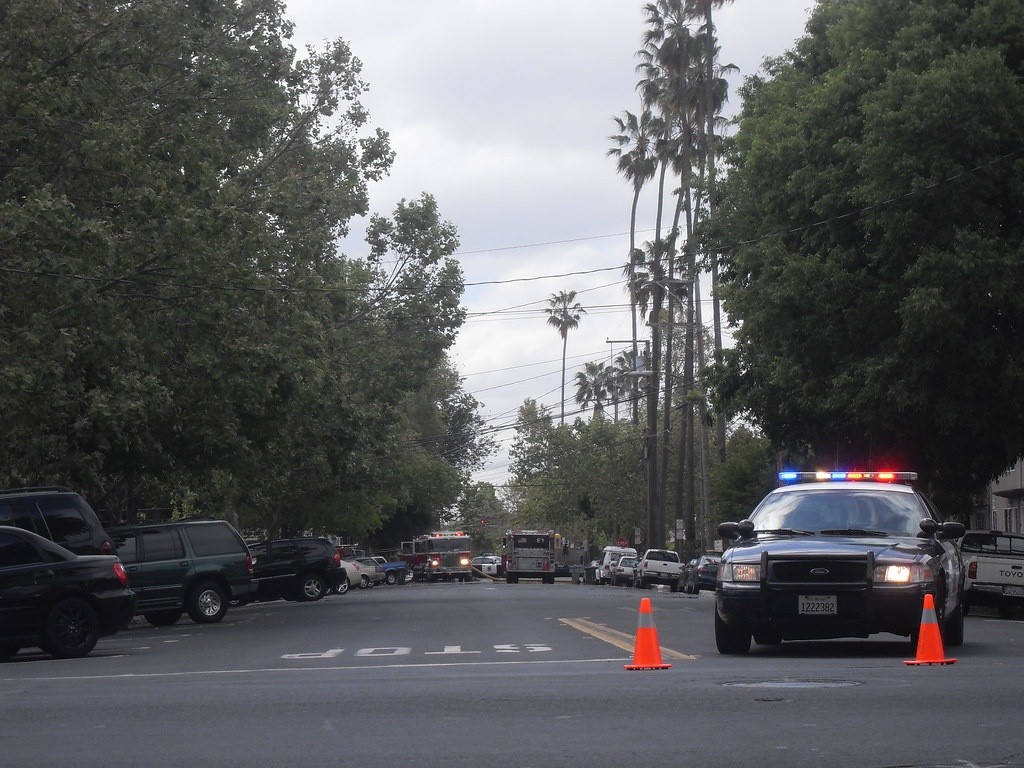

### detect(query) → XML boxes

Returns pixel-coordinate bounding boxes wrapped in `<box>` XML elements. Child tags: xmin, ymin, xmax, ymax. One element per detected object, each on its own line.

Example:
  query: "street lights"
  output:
<box><xmin>625</xmin><ymin>369</ymin><xmax>710</xmax><ymax>554</ymax></box>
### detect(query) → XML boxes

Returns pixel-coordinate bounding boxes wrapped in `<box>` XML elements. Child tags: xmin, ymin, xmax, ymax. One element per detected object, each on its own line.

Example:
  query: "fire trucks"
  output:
<box><xmin>502</xmin><ymin>528</ymin><xmax>555</xmax><ymax>585</ymax></box>
<box><xmin>402</xmin><ymin>531</ymin><xmax>472</xmax><ymax>584</ymax></box>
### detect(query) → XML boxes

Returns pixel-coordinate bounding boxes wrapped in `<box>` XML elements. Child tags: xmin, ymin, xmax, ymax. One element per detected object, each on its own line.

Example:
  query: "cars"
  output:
<box><xmin>471</xmin><ymin>553</ymin><xmax>502</xmax><ymax>578</ymax></box>
<box><xmin>611</xmin><ymin>556</ymin><xmax>641</xmax><ymax>586</ymax></box>
<box><xmin>0</xmin><ymin>526</ymin><xmax>136</xmax><ymax>661</ymax></box>
<box><xmin>582</xmin><ymin>560</ymin><xmax>604</xmax><ymax>585</ymax></box>
<box><xmin>331</xmin><ymin>545</ymin><xmax>407</xmax><ymax>594</ymax></box>
<box><xmin>716</xmin><ymin>472</ymin><xmax>967</xmax><ymax>656</ymax></box>
<box><xmin>678</xmin><ymin>555</ymin><xmax>721</xmax><ymax>593</ymax></box>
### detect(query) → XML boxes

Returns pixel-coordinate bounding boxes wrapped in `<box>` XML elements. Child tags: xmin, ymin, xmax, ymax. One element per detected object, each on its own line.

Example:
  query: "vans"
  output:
<box><xmin>599</xmin><ymin>544</ymin><xmax>637</xmax><ymax>585</ymax></box>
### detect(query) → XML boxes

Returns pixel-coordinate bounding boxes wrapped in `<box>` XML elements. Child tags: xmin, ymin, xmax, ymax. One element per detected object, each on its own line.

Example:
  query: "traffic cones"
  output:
<box><xmin>903</xmin><ymin>593</ymin><xmax>958</xmax><ymax>666</ymax></box>
<box><xmin>623</xmin><ymin>597</ymin><xmax>672</xmax><ymax>670</ymax></box>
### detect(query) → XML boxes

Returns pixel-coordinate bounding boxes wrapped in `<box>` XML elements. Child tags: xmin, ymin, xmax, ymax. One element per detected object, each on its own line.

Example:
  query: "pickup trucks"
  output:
<box><xmin>951</xmin><ymin>527</ymin><xmax>1024</xmax><ymax>606</ymax></box>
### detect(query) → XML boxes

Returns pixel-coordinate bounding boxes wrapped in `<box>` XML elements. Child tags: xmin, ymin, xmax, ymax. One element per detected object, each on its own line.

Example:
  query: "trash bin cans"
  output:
<box><xmin>583</xmin><ymin>566</ymin><xmax>598</xmax><ymax>584</ymax></box>
<box><xmin>570</xmin><ymin>565</ymin><xmax>581</xmax><ymax>585</ymax></box>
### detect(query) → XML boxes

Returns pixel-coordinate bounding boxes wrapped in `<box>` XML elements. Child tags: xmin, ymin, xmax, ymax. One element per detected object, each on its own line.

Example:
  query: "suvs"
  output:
<box><xmin>105</xmin><ymin>519</ymin><xmax>253</xmax><ymax>628</ymax></box>
<box><xmin>0</xmin><ymin>486</ymin><xmax>123</xmax><ymax>591</ymax></box>
<box><xmin>226</xmin><ymin>539</ymin><xmax>349</xmax><ymax>608</ymax></box>
<box><xmin>634</xmin><ymin>548</ymin><xmax>681</xmax><ymax>592</ymax></box>
